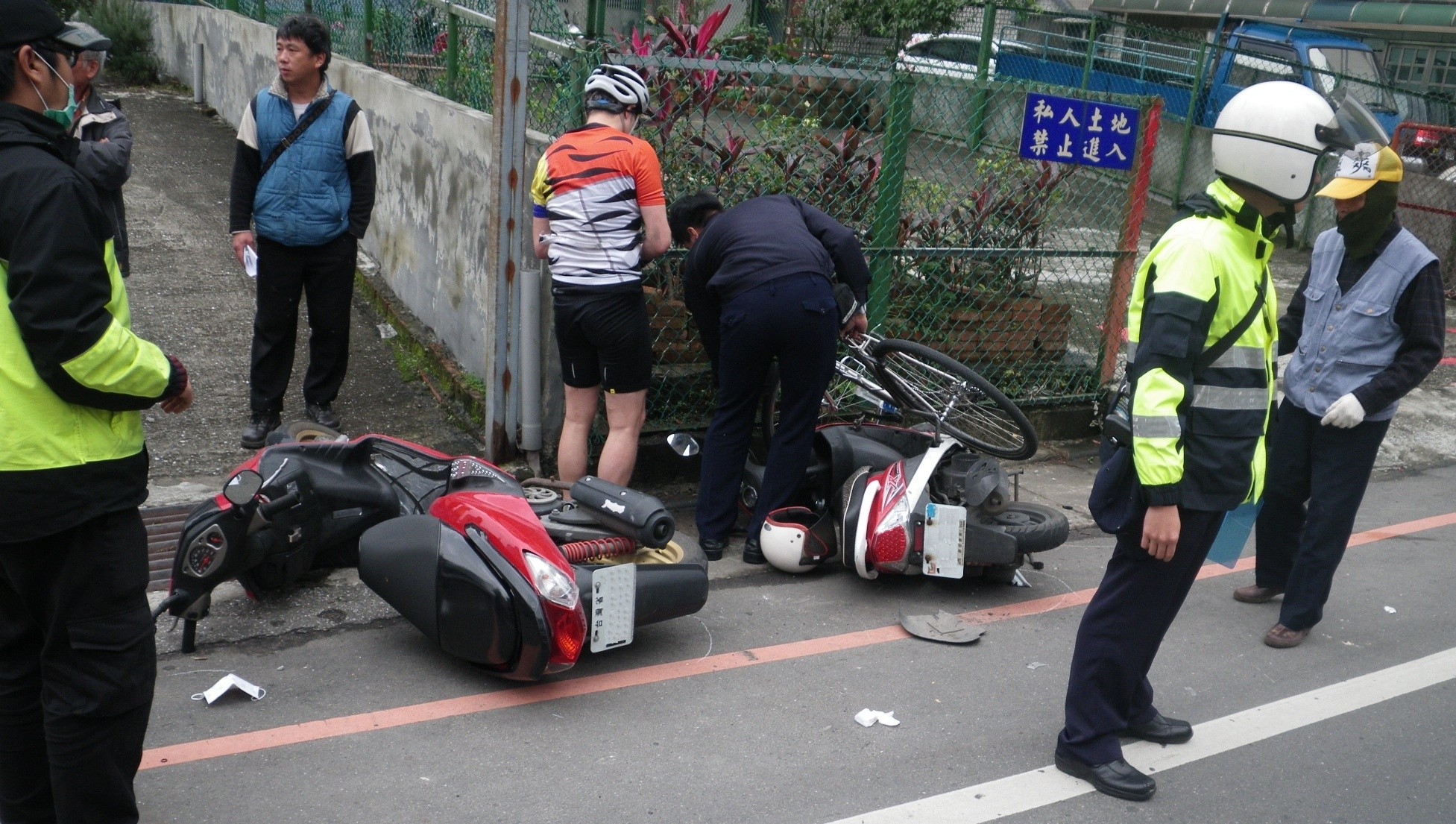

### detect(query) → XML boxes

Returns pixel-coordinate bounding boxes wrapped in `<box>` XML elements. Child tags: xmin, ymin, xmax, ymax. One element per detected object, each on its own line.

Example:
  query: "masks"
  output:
<box><xmin>42</xmin><ymin>84</ymin><xmax>78</xmax><ymax>130</ymax></box>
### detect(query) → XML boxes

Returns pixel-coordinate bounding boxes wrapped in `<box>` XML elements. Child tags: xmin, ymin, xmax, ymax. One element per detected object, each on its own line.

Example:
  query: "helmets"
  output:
<box><xmin>1212</xmin><ymin>80</ymin><xmax>1354</xmax><ymax>202</ymax></box>
<box><xmin>760</xmin><ymin>506</ymin><xmax>827</xmax><ymax>574</ymax></box>
<box><xmin>585</xmin><ymin>64</ymin><xmax>650</xmax><ymax>113</ymax></box>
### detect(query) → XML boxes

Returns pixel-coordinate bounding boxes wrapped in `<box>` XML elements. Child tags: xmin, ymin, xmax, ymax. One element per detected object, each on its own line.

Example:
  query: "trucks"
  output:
<box><xmin>995</xmin><ymin>13</ymin><xmax>1401</xmax><ymax>157</ymax></box>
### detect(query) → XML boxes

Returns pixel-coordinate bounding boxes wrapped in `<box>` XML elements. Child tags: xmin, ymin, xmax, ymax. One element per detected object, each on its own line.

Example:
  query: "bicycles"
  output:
<box><xmin>763</xmin><ymin>325</ymin><xmax>1040</xmax><ymax>462</ymax></box>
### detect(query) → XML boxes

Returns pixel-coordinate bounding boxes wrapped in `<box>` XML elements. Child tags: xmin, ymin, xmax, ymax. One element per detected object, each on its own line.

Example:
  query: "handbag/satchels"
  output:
<box><xmin>1088</xmin><ymin>391</ymin><xmax>1183</xmax><ymax>535</ymax></box>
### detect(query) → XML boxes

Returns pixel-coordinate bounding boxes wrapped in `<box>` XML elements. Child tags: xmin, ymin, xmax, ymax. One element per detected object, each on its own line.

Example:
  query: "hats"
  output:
<box><xmin>1313</xmin><ymin>141</ymin><xmax>1403</xmax><ymax>199</ymax></box>
<box><xmin>59</xmin><ymin>20</ymin><xmax>114</xmax><ymax>51</ymax></box>
<box><xmin>0</xmin><ymin>0</ymin><xmax>78</xmax><ymax>55</ymax></box>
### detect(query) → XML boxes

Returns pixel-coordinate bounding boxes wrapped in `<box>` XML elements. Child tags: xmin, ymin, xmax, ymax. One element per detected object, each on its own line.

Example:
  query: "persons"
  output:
<box><xmin>1054</xmin><ymin>81</ymin><xmax>1391</xmax><ymax>800</ymax></box>
<box><xmin>669</xmin><ymin>193</ymin><xmax>872</xmax><ymax>564</ymax></box>
<box><xmin>228</xmin><ymin>15</ymin><xmax>376</xmax><ymax>449</ymax></box>
<box><xmin>0</xmin><ymin>0</ymin><xmax>195</xmax><ymax>824</ymax></box>
<box><xmin>530</xmin><ymin>63</ymin><xmax>672</xmax><ymax>502</ymax></box>
<box><xmin>1234</xmin><ymin>143</ymin><xmax>1446</xmax><ymax>648</ymax></box>
<box><xmin>61</xmin><ymin>21</ymin><xmax>133</xmax><ymax>278</ymax></box>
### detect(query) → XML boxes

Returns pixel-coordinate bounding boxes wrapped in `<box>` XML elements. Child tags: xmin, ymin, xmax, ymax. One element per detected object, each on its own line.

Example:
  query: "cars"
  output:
<box><xmin>892</xmin><ymin>34</ymin><xmax>1033</xmax><ymax>86</ymax></box>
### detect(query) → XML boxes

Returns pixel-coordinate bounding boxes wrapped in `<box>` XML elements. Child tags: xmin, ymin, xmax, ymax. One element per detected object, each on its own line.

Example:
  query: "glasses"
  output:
<box><xmin>40</xmin><ymin>40</ymin><xmax>79</xmax><ymax>68</ymax></box>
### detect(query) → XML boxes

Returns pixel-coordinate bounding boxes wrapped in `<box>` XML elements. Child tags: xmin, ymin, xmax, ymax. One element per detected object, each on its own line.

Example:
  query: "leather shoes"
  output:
<box><xmin>240</xmin><ymin>414</ymin><xmax>282</xmax><ymax>448</ymax></box>
<box><xmin>1266</xmin><ymin>622</ymin><xmax>1312</xmax><ymax>648</ymax></box>
<box><xmin>1125</xmin><ymin>713</ymin><xmax>1194</xmax><ymax>744</ymax></box>
<box><xmin>744</xmin><ymin>537</ymin><xmax>768</xmax><ymax>565</ymax></box>
<box><xmin>306</xmin><ymin>402</ymin><xmax>342</xmax><ymax>431</ymax></box>
<box><xmin>1056</xmin><ymin>757</ymin><xmax>1156</xmax><ymax>801</ymax></box>
<box><xmin>1233</xmin><ymin>585</ymin><xmax>1283</xmax><ymax>604</ymax></box>
<box><xmin>701</xmin><ymin>539</ymin><xmax>727</xmax><ymax>561</ymax></box>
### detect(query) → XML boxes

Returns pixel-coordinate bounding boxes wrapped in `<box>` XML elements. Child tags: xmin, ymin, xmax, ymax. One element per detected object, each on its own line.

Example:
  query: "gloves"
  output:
<box><xmin>1321</xmin><ymin>392</ymin><xmax>1366</xmax><ymax>429</ymax></box>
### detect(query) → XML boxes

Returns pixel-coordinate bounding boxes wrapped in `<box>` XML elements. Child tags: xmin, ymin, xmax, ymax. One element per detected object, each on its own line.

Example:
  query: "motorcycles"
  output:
<box><xmin>152</xmin><ymin>420</ymin><xmax>709</xmax><ymax>684</ymax></box>
<box><xmin>665</xmin><ymin>411</ymin><xmax>1070</xmax><ymax>587</ymax></box>
